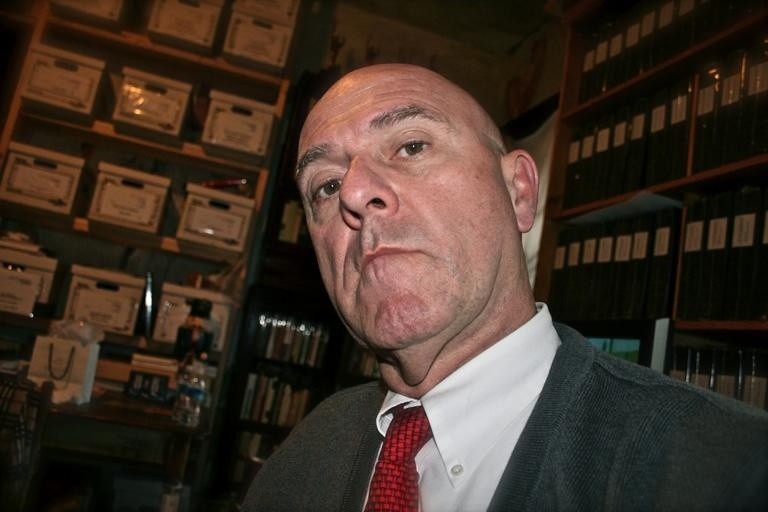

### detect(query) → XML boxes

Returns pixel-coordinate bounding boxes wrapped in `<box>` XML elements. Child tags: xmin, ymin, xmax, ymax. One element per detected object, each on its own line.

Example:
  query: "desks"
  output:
<box><xmin>18</xmin><ymin>376</ymin><xmax>200</xmax><ymax>512</ymax></box>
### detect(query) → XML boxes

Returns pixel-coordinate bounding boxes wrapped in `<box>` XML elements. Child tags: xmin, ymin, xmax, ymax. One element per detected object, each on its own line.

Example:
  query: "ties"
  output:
<box><xmin>365</xmin><ymin>406</ymin><xmax>432</xmax><ymax>511</ymax></box>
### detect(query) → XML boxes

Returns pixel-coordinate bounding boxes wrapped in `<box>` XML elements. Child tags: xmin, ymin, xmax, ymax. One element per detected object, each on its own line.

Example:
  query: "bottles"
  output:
<box><xmin>168</xmin><ymin>296</ymin><xmax>214</xmax><ymax>429</ymax></box>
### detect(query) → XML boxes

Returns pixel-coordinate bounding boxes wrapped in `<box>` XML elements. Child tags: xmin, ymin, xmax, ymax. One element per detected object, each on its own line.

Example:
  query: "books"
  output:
<box><xmin>548</xmin><ymin>0</ymin><xmax>766</xmax><ymax>409</ymax></box>
<box><xmin>277</xmin><ymin>198</ymin><xmax>311</xmax><ymax>245</ymax></box>
<box><xmin>227</xmin><ymin>311</ymin><xmax>382</xmax><ymax>501</ymax></box>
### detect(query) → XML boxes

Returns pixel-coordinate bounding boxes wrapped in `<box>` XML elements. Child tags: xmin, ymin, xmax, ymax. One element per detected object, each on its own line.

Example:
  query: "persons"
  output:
<box><xmin>232</xmin><ymin>59</ymin><xmax>766</xmax><ymax>511</ymax></box>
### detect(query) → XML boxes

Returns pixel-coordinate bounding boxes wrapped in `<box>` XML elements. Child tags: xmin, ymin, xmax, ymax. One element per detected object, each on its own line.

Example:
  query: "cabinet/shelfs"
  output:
<box><xmin>532</xmin><ymin>6</ymin><xmax>765</xmax><ymax>335</ymax></box>
<box><xmin>209</xmin><ymin>92</ymin><xmax>381</xmax><ymax>512</ymax></box>
<box><xmin>1</xmin><ymin>13</ymin><xmax>292</xmax><ymax>512</ymax></box>
